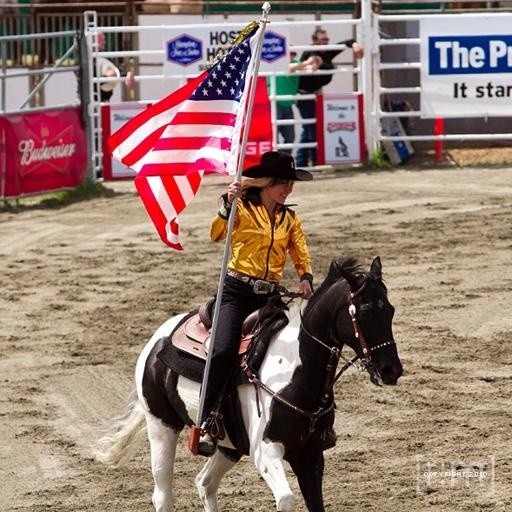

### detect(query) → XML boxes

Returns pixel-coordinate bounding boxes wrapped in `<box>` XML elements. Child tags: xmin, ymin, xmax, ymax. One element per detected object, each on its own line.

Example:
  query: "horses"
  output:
<box><xmin>93</xmin><ymin>250</ymin><xmax>403</xmax><ymax>512</ymax></box>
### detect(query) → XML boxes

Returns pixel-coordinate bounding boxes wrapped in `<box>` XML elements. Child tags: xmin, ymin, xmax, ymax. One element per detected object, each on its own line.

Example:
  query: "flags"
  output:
<box><xmin>106</xmin><ymin>18</ymin><xmax>262</xmax><ymax>250</ymax></box>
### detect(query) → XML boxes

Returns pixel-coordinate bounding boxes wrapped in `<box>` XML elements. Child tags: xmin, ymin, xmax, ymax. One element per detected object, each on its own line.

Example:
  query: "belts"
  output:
<box><xmin>225</xmin><ymin>269</ymin><xmax>281</xmax><ymax>296</ymax></box>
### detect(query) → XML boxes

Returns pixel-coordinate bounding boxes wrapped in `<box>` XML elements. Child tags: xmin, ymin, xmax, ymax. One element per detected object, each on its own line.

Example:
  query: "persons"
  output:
<box><xmin>73</xmin><ymin>30</ymin><xmax>117</xmax><ymax>178</ymax></box>
<box><xmin>266</xmin><ymin>44</ymin><xmax>323</xmax><ymax>154</ymax></box>
<box><xmin>198</xmin><ymin>152</ymin><xmax>313</xmax><ymax>456</ymax></box>
<box><xmin>295</xmin><ymin>30</ymin><xmax>363</xmax><ymax>168</ymax></box>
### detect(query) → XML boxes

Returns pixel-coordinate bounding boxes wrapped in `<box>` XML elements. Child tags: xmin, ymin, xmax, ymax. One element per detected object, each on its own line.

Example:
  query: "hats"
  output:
<box><xmin>96</xmin><ymin>58</ymin><xmax>121</xmax><ymax>92</ymax></box>
<box><xmin>241</xmin><ymin>151</ymin><xmax>313</xmax><ymax>181</ymax></box>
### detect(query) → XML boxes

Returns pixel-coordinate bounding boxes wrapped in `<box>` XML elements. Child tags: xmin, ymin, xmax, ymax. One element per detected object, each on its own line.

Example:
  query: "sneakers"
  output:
<box><xmin>198</xmin><ymin>420</ymin><xmax>218</xmax><ymax>454</ymax></box>
